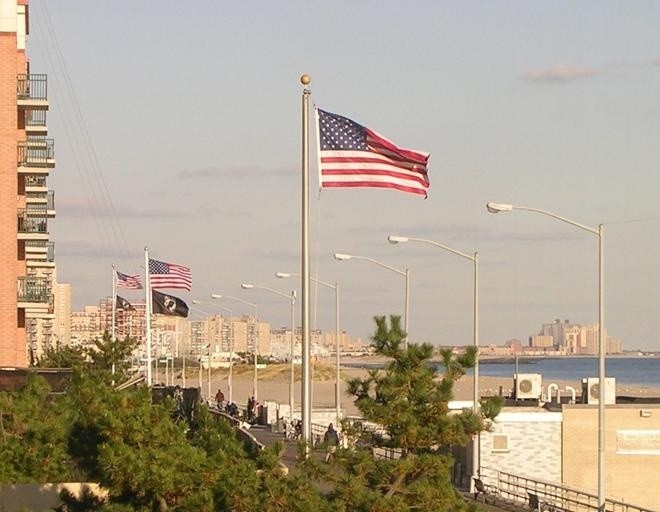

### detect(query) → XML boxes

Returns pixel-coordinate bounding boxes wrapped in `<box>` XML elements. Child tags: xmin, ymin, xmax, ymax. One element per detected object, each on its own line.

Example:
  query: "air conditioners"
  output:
<box><xmin>587</xmin><ymin>377</ymin><xmax>616</xmax><ymax>405</ymax></box>
<box><xmin>513</xmin><ymin>373</ymin><xmax>542</xmax><ymax>400</ymax></box>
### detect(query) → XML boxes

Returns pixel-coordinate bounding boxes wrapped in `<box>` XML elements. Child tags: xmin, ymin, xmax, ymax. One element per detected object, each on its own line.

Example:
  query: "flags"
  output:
<box><xmin>152</xmin><ymin>289</ymin><xmax>189</xmax><ymax>317</ymax></box>
<box><xmin>148</xmin><ymin>258</ymin><xmax>192</xmax><ymax>290</ymax></box>
<box><xmin>115</xmin><ymin>270</ymin><xmax>144</xmax><ymax>290</ymax></box>
<box><xmin>316</xmin><ymin>109</ymin><xmax>430</xmax><ymax>199</ymax></box>
<box><xmin>116</xmin><ymin>296</ymin><xmax>135</xmax><ymax>311</ymax></box>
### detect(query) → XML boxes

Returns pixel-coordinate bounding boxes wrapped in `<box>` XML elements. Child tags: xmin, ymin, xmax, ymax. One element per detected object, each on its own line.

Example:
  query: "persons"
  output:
<box><xmin>214</xmin><ymin>390</ymin><xmax>340</xmax><ymax>465</ymax></box>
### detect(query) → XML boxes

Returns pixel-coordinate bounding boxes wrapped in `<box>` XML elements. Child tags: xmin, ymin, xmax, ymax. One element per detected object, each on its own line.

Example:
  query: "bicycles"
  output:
<box><xmin>287</xmin><ymin>427</ymin><xmax>304</xmax><ymax>446</ymax></box>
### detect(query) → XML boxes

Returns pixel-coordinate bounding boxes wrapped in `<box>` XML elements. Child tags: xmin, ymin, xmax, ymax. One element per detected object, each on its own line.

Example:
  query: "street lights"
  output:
<box><xmin>156</xmin><ymin>272</ymin><xmax>340</xmax><ymax>427</ymax></box>
<box><xmin>487</xmin><ymin>203</ymin><xmax>606</xmax><ymax>512</ymax></box>
<box><xmin>388</xmin><ymin>234</ymin><xmax>478</xmax><ymax>415</ymax></box>
<box><xmin>334</xmin><ymin>252</ymin><xmax>408</xmax><ymax>350</ymax></box>
